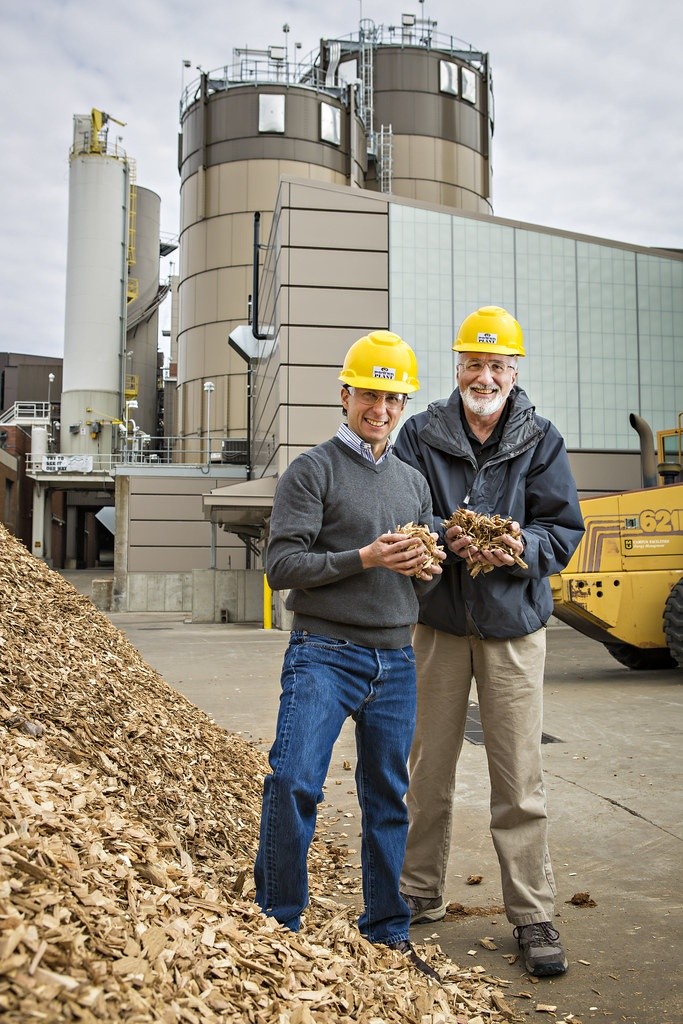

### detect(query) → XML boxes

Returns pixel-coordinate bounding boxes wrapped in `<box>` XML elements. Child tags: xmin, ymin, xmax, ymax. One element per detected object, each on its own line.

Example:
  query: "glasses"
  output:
<box><xmin>457</xmin><ymin>358</ymin><xmax>515</xmax><ymax>374</ymax></box>
<box><xmin>347</xmin><ymin>386</ymin><xmax>412</xmax><ymax>408</ymax></box>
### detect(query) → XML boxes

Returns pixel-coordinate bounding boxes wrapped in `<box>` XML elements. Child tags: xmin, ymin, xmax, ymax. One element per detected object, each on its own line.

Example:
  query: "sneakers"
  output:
<box><xmin>513</xmin><ymin>919</ymin><xmax>569</xmax><ymax>977</ymax></box>
<box><xmin>398</xmin><ymin>892</ymin><xmax>446</xmax><ymax>925</ymax></box>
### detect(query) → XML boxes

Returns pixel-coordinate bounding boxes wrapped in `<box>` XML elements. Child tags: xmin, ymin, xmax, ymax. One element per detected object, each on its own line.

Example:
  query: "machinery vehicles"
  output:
<box><xmin>544</xmin><ymin>406</ymin><xmax>683</xmax><ymax>674</ymax></box>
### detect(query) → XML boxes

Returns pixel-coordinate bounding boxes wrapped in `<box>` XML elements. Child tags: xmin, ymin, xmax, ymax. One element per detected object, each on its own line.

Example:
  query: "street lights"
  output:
<box><xmin>204</xmin><ymin>379</ymin><xmax>214</xmax><ymax>466</ymax></box>
<box><xmin>47</xmin><ymin>373</ymin><xmax>55</xmax><ymax>425</ymax></box>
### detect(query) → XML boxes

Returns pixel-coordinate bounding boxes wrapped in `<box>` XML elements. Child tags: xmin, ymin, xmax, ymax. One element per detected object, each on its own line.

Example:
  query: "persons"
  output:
<box><xmin>400</xmin><ymin>304</ymin><xmax>587</xmax><ymax>977</ymax></box>
<box><xmin>253</xmin><ymin>329</ymin><xmax>447</xmax><ymax>979</ymax></box>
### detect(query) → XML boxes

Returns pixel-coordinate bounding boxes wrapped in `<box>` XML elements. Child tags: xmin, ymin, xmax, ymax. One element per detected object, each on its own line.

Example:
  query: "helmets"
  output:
<box><xmin>452</xmin><ymin>306</ymin><xmax>525</xmax><ymax>358</ymax></box>
<box><xmin>337</xmin><ymin>330</ymin><xmax>419</xmax><ymax>393</ymax></box>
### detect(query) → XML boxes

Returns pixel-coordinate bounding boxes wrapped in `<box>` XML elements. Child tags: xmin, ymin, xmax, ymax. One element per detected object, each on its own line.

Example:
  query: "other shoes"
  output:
<box><xmin>392</xmin><ymin>941</ymin><xmax>441</xmax><ymax>984</ymax></box>
